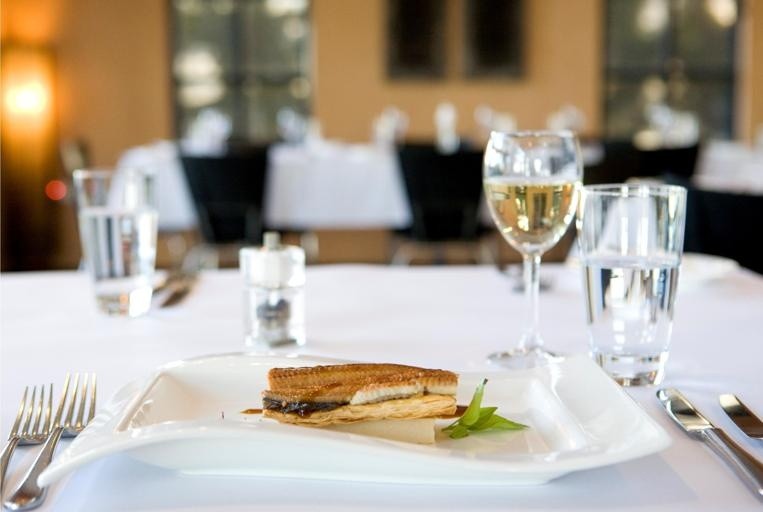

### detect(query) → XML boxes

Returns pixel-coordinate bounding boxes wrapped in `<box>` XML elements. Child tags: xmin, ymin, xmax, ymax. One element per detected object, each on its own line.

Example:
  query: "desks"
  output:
<box><xmin>0</xmin><ymin>253</ymin><xmax>763</xmax><ymax>510</ymax></box>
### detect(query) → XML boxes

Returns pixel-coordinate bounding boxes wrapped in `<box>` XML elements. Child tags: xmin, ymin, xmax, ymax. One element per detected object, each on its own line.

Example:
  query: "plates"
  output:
<box><xmin>36</xmin><ymin>353</ymin><xmax>674</xmax><ymax>486</ymax></box>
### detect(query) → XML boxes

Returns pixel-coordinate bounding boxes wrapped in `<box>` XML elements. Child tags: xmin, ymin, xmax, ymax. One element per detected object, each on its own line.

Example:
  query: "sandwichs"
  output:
<box><xmin>261</xmin><ymin>363</ymin><xmax>459</xmax><ymax>426</ymax></box>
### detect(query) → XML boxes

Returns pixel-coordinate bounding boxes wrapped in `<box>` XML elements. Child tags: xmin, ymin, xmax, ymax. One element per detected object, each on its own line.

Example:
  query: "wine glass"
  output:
<box><xmin>482</xmin><ymin>128</ymin><xmax>584</xmax><ymax>367</ymax></box>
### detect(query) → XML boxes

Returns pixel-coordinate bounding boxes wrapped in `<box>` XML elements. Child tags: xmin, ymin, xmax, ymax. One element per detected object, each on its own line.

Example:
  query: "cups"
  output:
<box><xmin>71</xmin><ymin>165</ymin><xmax>160</xmax><ymax>319</ymax></box>
<box><xmin>578</xmin><ymin>182</ymin><xmax>687</xmax><ymax>387</ymax></box>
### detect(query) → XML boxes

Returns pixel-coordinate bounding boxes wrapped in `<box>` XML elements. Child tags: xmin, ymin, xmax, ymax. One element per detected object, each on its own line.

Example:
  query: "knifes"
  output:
<box><xmin>718</xmin><ymin>394</ymin><xmax>762</xmax><ymax>443</ymax></box>
<box><xmin>656</xmin><ymin>387</ymin><xmax>762</xmax><ymax>506</ymax></box>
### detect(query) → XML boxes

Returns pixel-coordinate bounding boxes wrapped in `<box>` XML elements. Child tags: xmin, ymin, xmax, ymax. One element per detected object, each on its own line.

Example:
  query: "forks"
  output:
<box><xmin>3</xmin><ymin>372</ymin><xmax>96</xmax><ymax>512</ymax></box>
<box><xmin>0</xmin><ymin>383</ymin><xmax>52</xmax><ymax>494</ymax></box>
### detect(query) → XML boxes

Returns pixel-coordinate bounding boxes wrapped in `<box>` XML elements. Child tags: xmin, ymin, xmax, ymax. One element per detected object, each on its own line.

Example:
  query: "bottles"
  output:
<box><xmin>238</xmin><ymin>233</ymin><xmax>306</xmax><ymax>355</ymax></box>
